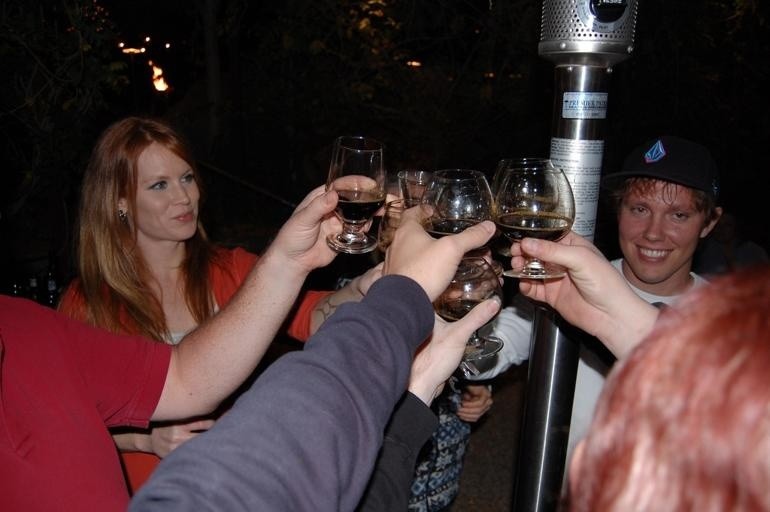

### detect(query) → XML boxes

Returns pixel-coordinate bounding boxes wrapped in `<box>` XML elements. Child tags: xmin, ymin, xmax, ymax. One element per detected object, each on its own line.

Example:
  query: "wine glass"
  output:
<box><xmin>494</xmin><ymin>160</ymin><xmax>556</xmax><ymax>256</ymax></box>
<box><xmin>377</xmin><ymin>198</ymin><xmax>418</xmax><ymax>252</ymax></box>
<box><xmin>433</xmin><ymin>257</ymin><xmax>503</xmax><ymax>361</ymax></box>
<box><xmin>420</xmin><ymin>169</ymin><xmax>496</xmax><ymax>281</ymax></box>
<box><xmin>499</xmin><ymin>168</ymin><xmax>575</xmax><ymax>281</ymax></box>
<box><xmin>324</xmin><ymin>136</ymin><xmax>388</xmax><ymax>256</ymax></box>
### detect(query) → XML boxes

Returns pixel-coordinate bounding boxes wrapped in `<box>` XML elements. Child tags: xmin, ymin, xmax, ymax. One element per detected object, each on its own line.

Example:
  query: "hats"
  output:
<box><xmin>600</xmin><ymin>127</ymin><xmax>723</xmax><ymax>191</ymax></box>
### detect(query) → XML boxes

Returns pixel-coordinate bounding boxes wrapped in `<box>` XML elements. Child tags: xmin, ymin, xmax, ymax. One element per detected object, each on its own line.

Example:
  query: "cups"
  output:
<box><xmin>399</xmin><ymin>172</ymin><xmax>429</xmax><ymax>210</ymax></box>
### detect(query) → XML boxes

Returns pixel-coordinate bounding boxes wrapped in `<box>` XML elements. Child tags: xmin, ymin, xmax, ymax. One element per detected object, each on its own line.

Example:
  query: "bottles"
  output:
<box><xmin>2</xmin><ymin>271</ymin><xmax>61</xmax><ymax>311</ymax></box>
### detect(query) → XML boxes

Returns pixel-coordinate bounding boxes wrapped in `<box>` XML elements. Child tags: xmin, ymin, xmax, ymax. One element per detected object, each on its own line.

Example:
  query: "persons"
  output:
<box><xmin>1</xmin><ymin>118</ymin><xmax>768</xmax><ymax>512</ymax></box>
